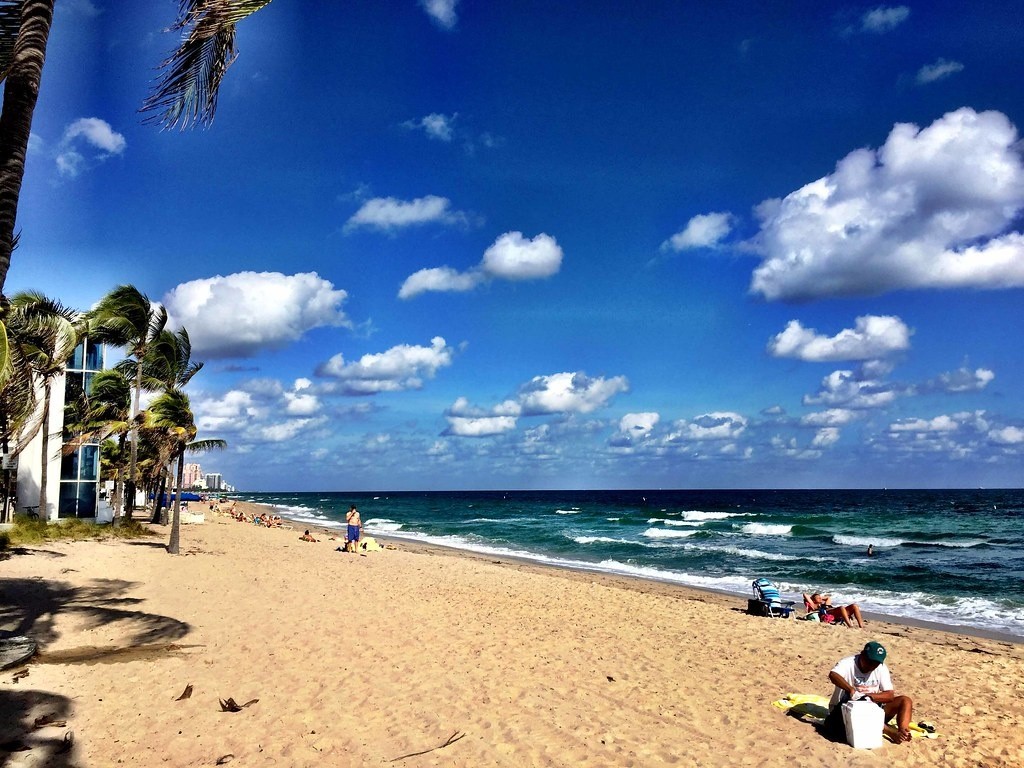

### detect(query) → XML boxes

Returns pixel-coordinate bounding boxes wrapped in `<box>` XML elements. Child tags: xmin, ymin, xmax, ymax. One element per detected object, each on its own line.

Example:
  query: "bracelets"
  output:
<box><xmin>359</xmin><ymin>527</ymin><xmax>362</xmax><ymax>528</ymax></box>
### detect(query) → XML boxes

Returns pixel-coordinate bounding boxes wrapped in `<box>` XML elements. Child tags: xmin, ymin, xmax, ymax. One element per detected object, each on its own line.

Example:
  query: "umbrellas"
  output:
<box><xmin>148</xmin><ymin>492</ymin><xmax>200</xmax><ymax>510</ymax></box>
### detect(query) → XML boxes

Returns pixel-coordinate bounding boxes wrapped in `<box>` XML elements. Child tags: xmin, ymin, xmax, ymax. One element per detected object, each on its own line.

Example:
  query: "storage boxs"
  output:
<box><xmin>841</xmin><ymin>699</ymin><xmax>886</xmax><ymax>750</ymax></box>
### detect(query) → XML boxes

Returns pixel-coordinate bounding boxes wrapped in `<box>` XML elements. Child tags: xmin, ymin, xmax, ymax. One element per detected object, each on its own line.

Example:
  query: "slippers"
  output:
<box><xmin>917</xmin><ymin>721</ymin><xmax>936</xmax><ymax>733</ymax></box>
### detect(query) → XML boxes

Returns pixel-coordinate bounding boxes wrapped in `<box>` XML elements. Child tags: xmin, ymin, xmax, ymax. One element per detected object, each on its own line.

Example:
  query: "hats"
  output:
<box><xmin>864</xmin><ymin>641</ymin><xmax>887</xmax><ymax>666</ymax></box>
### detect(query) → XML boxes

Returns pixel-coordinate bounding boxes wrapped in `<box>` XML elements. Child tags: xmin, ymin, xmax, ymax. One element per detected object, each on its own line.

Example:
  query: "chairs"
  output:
<box><xmin>804</xmin><ymin>597</ymin><xmax>853</xmax><ymax>626</ymax></box>
<box><xmin>161</xmin><ymin>506</ymin><xmax>205</xmax><ymax>525</ymax></box>
<box><xmin>752</xmin><ymin>577</ymin><xmax>796</xmax><ymax>619</ymax></box>
<box><xmin>212</xmin><ymin>503</ymin><xmax>293</xmax><ymax>531</ymax></box>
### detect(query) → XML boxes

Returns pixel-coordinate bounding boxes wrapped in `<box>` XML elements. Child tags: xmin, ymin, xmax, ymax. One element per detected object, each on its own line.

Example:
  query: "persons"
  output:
<box><xmin>828</xmin><ymin>641</ymin><xmax>913</xmax><ymax>745</ymax></box>
<box><xmin>803</xmin><ymin>592</ymin><xmax>865</xmax><ymax>629</ymax></box>
<box><xmin>868</xmin><ymin>544</ymin><xmax>873</xmax><ymax>557</ymax></box>
<box><xmin>200</xmin><ymin>493</ymin><xmax>282</xmax><ymax>528</ymax></box>
<box><xmin>302</xmin><ymin>530</ymin><xmax>316</xmax><ymax>542</ymax></box>
<box><xmin>345</xmin><ymin>504</ymin><xmax>364</xmax><ymax>554</ymax></box>
